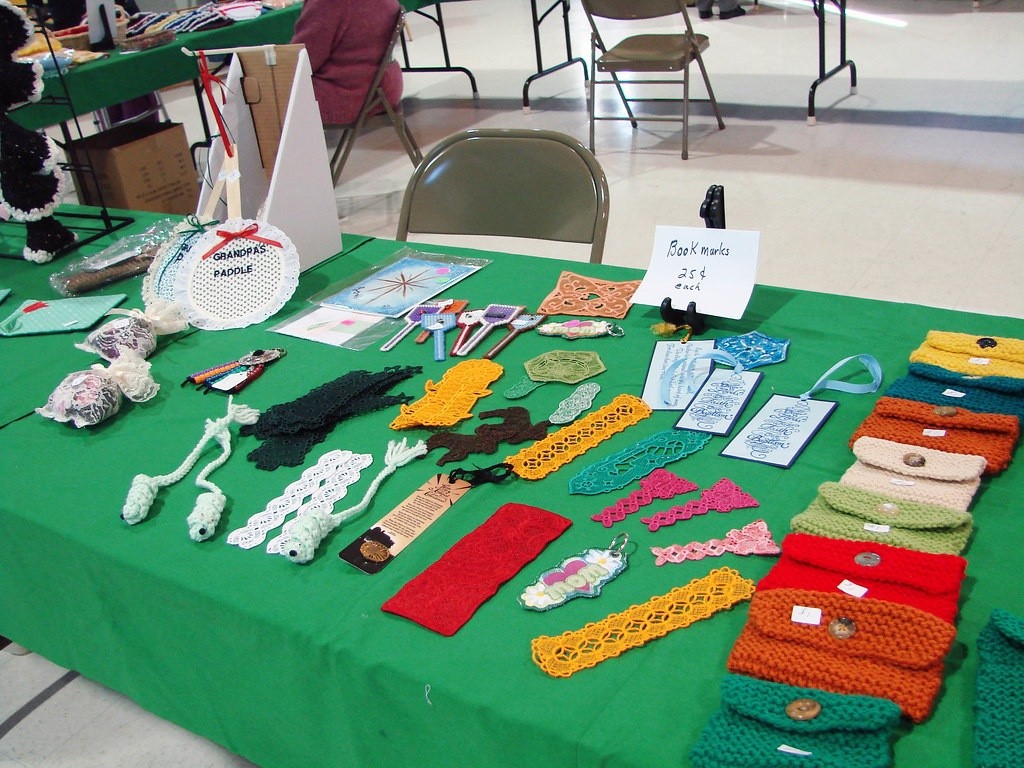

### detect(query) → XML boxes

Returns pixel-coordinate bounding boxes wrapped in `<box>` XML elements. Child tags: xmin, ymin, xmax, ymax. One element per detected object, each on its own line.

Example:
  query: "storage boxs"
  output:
<box><xmin>54</xmin><ymin>20</ymin><xmax>126</xmax><ymax>50</ymax></box>
<box><xmin>61</xmin><ymin>121</ymin><xmax>199</xmax><ymax>214</ymax></box>
<box><xmin>195</xmin><ymin>46</ymin><xmax>343</xmax><ymax>272</ymax></box>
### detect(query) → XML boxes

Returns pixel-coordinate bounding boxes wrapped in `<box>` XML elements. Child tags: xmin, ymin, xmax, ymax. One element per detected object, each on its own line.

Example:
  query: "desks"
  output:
<box><xmin>1</xmin><ymin>238</ymin><xmax>1024</xmax><ymax>768</ymax></box>
<box><xmin>5</xmin><ymin>0</ymin><xmax>479</xmax><ymax>216</ymax></box>
<box><xmin>523</xmin><ymin>0</ymin><xmax>857</xmax><ymax>126</ymax></box>
<box><xmin>0</xmin><ymin>202</ymin><xmax>373</xmax><ymax>435</ymax></box>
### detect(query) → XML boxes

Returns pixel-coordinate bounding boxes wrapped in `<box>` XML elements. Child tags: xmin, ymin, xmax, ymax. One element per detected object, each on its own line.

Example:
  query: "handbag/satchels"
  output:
<box><xmin>722</xmin><ymin>586</ymin><xmax>959</xmax><ymax>725</ymax></box>
<box><xmin>849</xmin><ymin>395</ymin><xmax>1021</xmax><ymax>478</ymax></box>
<box><xmin>879</xmin><ymin>361</ymin><xmax>1024</xmax><ymax>427</ymax></box>
<box><xmin>685</xmin><ymin>673</ymin><xmax>905</xmax><ymax>767</ymax></box>
<box><xmin>971</xmin><ymin>610</ymin><xmax>1024</xmax><ymax>768</ymax></box>
<box><xmin>908</xmin><ymin>330</ymin><xmax>1024</xmax><ymax>380</ymax></box>
<box><xmin>755</xmin><ymin>533</ymin><xmax>968</xmax><ymax>633</ymax></box>
<box><xmin>789</xmin><ymin>481</ymin><xmax>975</xmax><ymax>560</ymax></box>
<box><xmin>838</xmin><ymin>435</ymin><xmax>987</xmax><ymax>512</ymax></box>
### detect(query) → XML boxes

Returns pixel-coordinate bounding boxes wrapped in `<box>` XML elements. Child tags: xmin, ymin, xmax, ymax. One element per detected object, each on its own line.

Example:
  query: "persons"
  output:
<box><xmin>292</xmin><ymin>0</ymin><xmax>403</xmax><ymax>124</ymax></box>
<box><xmin>697</xmin><ymin>0</ymin><xmax>746</xmax><ymax>19</ymax></box>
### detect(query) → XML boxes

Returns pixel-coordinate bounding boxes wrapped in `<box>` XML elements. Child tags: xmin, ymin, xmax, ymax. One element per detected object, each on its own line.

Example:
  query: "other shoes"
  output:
<box><xmin>720</xmin><ymin>4</ymin><xmax>745</xmax><ymax>19</ymax></box>
<box><xmin>699</xmin><ymin>7</ymin><xmax>713</xmax><ymax>18</ymax></box>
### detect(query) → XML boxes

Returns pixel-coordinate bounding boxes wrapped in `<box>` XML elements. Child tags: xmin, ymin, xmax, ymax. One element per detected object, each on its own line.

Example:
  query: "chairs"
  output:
<box><xmin>330</xmin><ymin>6</ymin><xmax>424</xmax><ymax>186</ymax></box>
<box><xmin>583</xmin><ymin>1</ymin><xmax>725</xmax><ymax>160</ymax></box>
<box><xmin>396</xmin><ymin>128</ymin><xmax>608</xmax><ymax>264</ymax></box>
